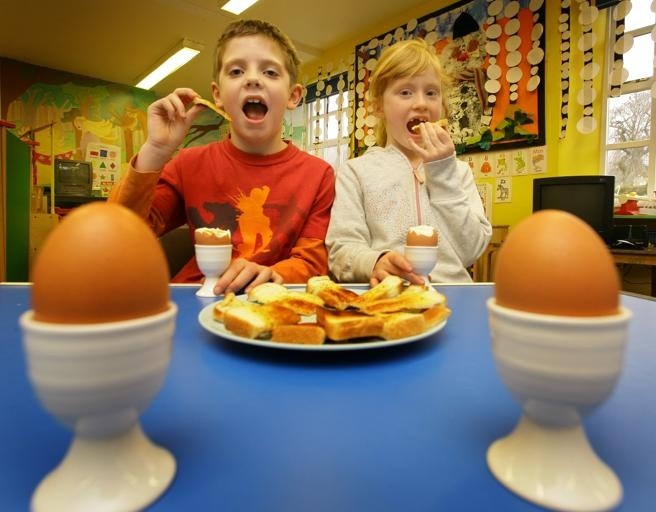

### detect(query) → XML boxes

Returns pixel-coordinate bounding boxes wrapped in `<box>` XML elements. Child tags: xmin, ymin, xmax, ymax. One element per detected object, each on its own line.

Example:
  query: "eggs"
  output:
<box><xmin>29</xmin><ymin>202</ymin><xmax>170</xmax><ymax>325</ymax></box>
<box><xmin>195</xmin><ymin>226</ymin><xmax>232</xmax><ymax>246</ymax></box>
<box><xmin>406</xmin><ymin>225</ymin><xmax>438</xmax><ymax>246</ymax></box>
<box><xmin>496</xmin><ymin>208</ymin><xmax>620</xmax><ymax>318</ymax></box>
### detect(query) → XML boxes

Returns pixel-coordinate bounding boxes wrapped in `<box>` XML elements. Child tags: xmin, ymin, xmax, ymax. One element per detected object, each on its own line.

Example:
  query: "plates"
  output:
<box><xmin>196</xmin><ymin>287</ymin><xmax>450</xmax><ymax>353</ymax></box>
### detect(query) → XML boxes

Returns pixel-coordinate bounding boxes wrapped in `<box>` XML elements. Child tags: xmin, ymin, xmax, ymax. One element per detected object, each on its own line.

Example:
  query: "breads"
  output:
<box><xmin>411</xmin><ymin>119</ymin><xmax>448</xmax><ymax>133</ymax></box>
<box><xmin>212</xmin><ymin>275</ymin><xmax>450</xmax><ymax>344</ymax></box>
<box><xmin>192</xmin><ymin>98</ymin><xmax>233</xmax><ymax>122</ymax></box>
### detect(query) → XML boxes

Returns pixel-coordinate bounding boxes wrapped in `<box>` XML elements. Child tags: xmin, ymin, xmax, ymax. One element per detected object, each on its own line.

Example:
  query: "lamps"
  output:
<box><xmin>133</xmin><ymin>39</ymin><xmax>205</xmax><ymax>91</ymax></box>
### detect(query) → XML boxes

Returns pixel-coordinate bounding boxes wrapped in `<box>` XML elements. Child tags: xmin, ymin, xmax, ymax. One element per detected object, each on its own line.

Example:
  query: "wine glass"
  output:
<box><xmin>17</xmin><ymin>299</ymin><xmax>181</xmax><ymax>512</ymax></box>
<box><xmin>193</xmin><ymin>244</ymin><xmax>234</xmax><ymax>298</ymax></box>
<box><xmin>404</xmin><ymin>245</ymin><xmax>439</xmax><ymax>292</ymax></box>
<box><xmin>483</xmin><ymin>296</ymin><xmax>634</xmax><ymax>512</ymax></box>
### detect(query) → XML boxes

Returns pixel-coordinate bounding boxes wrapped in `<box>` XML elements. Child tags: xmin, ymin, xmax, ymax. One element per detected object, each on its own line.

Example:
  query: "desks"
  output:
<box><xmin>481</xmin><ymin>241</ymin><xmax>655</xmax><ymax>284</ymax></box>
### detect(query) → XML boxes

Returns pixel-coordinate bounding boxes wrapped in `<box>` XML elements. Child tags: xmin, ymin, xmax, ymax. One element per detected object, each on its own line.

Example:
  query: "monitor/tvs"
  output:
<box><xmin>53</xmin><ymin>158</ymin><xmax>93</xmax><ymax>197</ymax></box>
<box><xmin>532</xmin><ymin>175</ymin><xmax>616</xmax><ymax>244</ymax></box>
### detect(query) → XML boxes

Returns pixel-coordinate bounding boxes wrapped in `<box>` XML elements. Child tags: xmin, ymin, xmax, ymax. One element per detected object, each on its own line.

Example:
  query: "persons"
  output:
<box><xmin>325</xmin><ymin>36</ymin><xmax>494</xmax><ymax>289</ymax></box>
<box><xmin>105</xmin><ymin>17</ymin><xmax>336</xmax><ymax>296</ymax></box>
